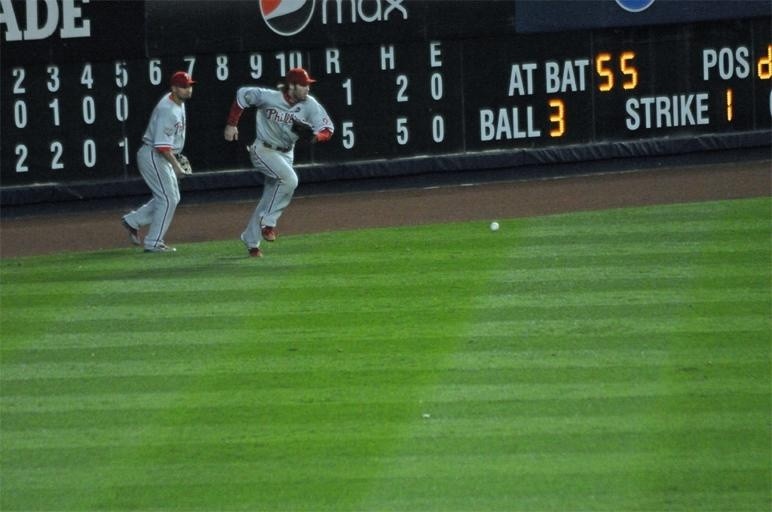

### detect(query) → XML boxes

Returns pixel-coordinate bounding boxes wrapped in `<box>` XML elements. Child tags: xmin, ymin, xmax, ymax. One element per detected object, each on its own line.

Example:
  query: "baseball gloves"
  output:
<box><xmin>290</xmin><ymin>117</ymin><xmax>317</xmax><ymax>147</ymax></box>
<box><xmin>175</xmin><ymin>155</ymin><xmax>192</xmax><ymax>177</ymax></box>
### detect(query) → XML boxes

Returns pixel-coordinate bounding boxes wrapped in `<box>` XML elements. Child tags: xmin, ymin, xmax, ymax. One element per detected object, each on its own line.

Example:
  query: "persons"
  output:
<box><xmin>223</xmin><ymin>67</ymin><xmax>335</xmax><ymax>257</ymax></box>
<box><xmin>121</xmin><ymin>71</ymin><xmax>198</xmax><ymax>252</ymax></box>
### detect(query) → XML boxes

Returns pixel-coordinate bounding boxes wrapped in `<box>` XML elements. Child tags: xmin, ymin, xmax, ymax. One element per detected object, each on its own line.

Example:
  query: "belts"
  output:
<box><xmin>263</xmin><ymin>142</ymin><xmax>289</xmax><ymax>152</ymax></box>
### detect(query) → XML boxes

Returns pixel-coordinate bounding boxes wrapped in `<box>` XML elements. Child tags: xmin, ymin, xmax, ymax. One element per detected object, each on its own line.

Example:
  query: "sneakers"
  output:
<box><xmin>144</xmin><ymin>244</ymin><xmax>176</xmax><ymax>253</ymax></box>
<box><xmin>248</xmin><ymin>248</ymin><xmax>262</xmax><ymax>257</ymax></box>
<box><xmin>120</xmin><ymin>216</ymin><xmax>140</xmax><ymax>246</ymax></box>
<box><xmin>259</xmin><ymin>216</ymin><xmax>276</xmax><ymax>241</ymax></box>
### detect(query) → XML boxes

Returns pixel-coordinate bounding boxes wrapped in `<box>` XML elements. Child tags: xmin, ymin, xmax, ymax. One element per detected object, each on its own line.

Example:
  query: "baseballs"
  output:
<box><xmin>491</xmin><ymin>222</ymin><xmax>499</xmax><ymax>231</ymax></box>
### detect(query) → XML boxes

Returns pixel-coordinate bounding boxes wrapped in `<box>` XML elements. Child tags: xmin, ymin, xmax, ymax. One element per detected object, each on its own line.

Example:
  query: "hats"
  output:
<box><xmin>169</xmin><ymin>71</ymin><xmax>198</xmax><ymax>88</ymax></box>
<box><xmin>285</xmin><ymin>68</ymin><xmax>317</xmax><ymax>86</ymax></box>
